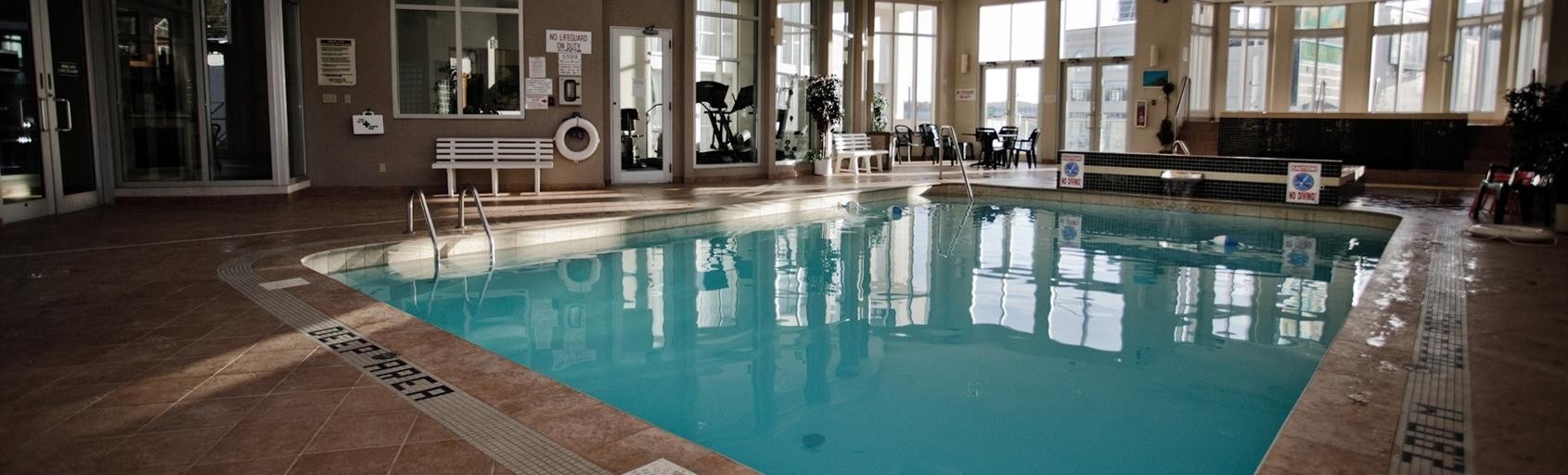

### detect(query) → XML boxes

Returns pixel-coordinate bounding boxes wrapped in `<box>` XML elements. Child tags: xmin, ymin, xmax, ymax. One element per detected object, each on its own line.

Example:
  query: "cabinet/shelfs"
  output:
<box><xmin>866</xmin><ymin>131</ymin><xmax>894</xmax><ymax>171</ymax></box>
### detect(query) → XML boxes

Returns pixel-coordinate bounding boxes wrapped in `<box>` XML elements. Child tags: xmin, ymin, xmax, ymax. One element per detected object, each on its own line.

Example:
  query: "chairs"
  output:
<box><xmin>895</xmin><ymin>124</ymin><xmax>1041</xmax><ymax>170</ymax></box>
<box><xmin>1471</xmin><ymin>147</ymin><xmax>1566</xmax><ymax>227</ymax></box>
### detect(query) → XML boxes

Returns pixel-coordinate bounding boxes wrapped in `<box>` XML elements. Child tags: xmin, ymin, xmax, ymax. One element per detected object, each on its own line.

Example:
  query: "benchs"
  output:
<box><xmin>832</xmin><ymin>132</ymin><xmax>889</xmax><ymax>173</ymax></box>
<box><xmin>431</xmin><ymin>136</ymin><xmax>556</xmax><ymax>197</ymax></box>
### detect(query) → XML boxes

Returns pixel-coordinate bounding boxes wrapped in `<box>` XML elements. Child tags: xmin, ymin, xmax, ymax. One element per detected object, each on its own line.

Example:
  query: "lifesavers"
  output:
<box><xmin>557</xmin><ymin>117</ymin><xmax>600</xmax><ymax>162</ymax></box>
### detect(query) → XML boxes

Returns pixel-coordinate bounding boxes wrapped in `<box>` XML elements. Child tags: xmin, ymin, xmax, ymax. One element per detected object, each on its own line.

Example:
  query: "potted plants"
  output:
<box><xmin>805</xmin><ymin>74</ymin><xmax>845</xmax><ymax>175</ymax></box>
